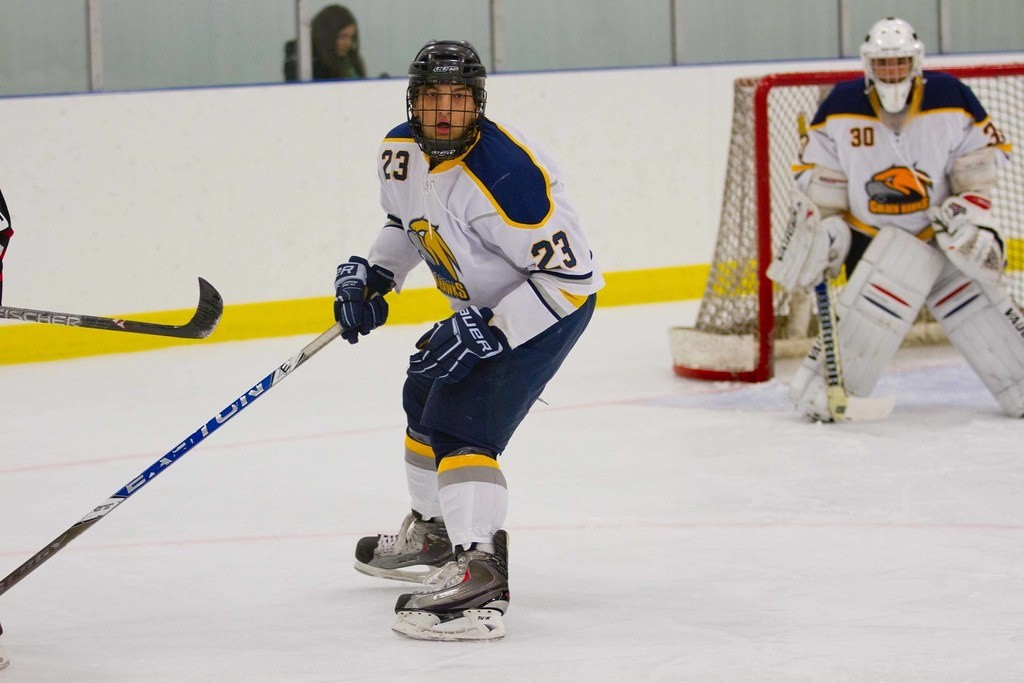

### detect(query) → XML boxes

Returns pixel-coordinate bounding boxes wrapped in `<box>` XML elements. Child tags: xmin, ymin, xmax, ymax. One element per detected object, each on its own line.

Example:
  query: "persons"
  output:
<box><xmin>766</xmin><ymin>17</ymin><xmax>1024</xmax><ymax>425</ymax></box>
<box><xmin>284</xmin><ymin>4</ymin><xmax>364</xmax><ymax>81</ymax></box>
<box><xmin>334</xmin><ymin>41</ymin><xmax>607</xmax><ymax>642</ymax></box>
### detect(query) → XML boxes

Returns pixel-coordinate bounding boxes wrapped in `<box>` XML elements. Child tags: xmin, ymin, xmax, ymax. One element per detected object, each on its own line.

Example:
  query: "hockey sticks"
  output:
<box><xmin>812</xmin><ymin>268</ymin><xmax>897</xmax><ymax>424</ymax></box>
<box><xmin>0</xmin><ymin>275</ymin><xmax>225</xmax><ymax>342</ymax></box>
<box><xmin>0</xmin><ymin>291</ymin><xmax>381</xmax><ymax>634</ymax></box>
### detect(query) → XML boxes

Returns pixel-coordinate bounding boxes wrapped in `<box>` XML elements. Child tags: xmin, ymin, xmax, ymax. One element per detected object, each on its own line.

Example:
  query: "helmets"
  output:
<box><xmin>859</xmin><ymin>16</ymin><xmax>925</xmax><ymax>113</ymax></box>
<box><xmin>405</xmin><ymin>39</ymin><xmax>488</xmax><ymax>160</ymax></box>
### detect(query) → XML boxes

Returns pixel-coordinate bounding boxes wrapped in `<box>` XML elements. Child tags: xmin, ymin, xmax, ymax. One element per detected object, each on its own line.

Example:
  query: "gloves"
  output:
<box><xmin>333</xmin><ymin>255</ymin><xmax>395</xmax><ymax>345</ymax></box>
<box><xmin>408</xmin><ymin>305</ymin><xmax>506</xmax><ymax>385</ymax></box>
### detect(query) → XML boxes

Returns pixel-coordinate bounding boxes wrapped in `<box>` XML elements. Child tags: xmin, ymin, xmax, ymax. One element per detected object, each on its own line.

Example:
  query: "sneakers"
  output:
<box><xmin>392</xmin><ymin>529</ymin><xmax>511</xmax><ymax>643</ymax></box>
<box><xmin>354</xmin><ymin>508</ymin><xmax>454</xmax><ymax>584</ymax></box>
